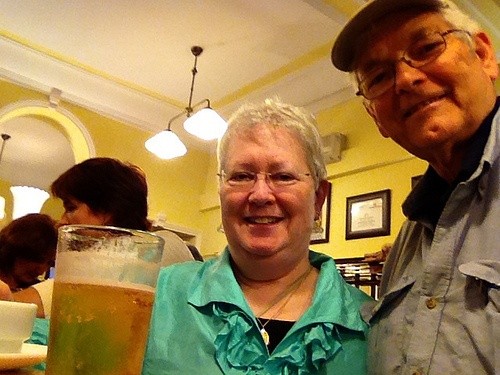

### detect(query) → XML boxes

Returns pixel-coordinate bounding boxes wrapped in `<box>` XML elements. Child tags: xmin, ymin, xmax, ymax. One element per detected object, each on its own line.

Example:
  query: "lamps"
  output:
<box><xmin>145</xmin><ymin>45</ymin><xmax>228</xmax><ymax>161</ymax></box>
<box><xmin>0</xmin><ymin>133</ymin><xmax>50</xmax><ymax>220</ymax></box>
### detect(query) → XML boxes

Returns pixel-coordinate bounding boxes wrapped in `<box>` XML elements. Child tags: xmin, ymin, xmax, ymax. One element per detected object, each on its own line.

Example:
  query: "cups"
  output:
<box><xmin>46</xmin><ymin>225</ymin><xmax>165</xmax><ymax>374</ymax></box>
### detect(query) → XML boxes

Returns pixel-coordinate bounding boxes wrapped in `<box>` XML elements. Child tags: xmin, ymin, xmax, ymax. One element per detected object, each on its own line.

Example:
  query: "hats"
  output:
<box><xmin>331</xmin><ymin>0</ymin><xmax>450</xmax><ymax>72</ymax></box>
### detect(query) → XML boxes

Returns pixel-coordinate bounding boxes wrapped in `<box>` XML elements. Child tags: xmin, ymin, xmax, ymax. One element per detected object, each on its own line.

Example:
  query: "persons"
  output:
<box><xmin>0</xmin><ymin>213</ymin><xmax>59</xmax><ymax>299</ymax></box>
<box><xmin>1</xmin><ymin>156</ymin><xmax>204</xmax><ymax>319</ymax></box>
<box><xmin>331</xmin><ymin>0</ymin><xmax>499</xmax><ymax>375</ymax></box>
<box><xmin>26</xmin><ymin>96</ymin><xmax>379</xmax><ymax>375</ymax></box>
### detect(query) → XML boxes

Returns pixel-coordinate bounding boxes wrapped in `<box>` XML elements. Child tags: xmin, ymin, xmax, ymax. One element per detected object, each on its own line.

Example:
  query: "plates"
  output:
<box><xmin>0</xmin><ymin>343</ymin><xmax>48</xmax><ymax>370</ymax></box>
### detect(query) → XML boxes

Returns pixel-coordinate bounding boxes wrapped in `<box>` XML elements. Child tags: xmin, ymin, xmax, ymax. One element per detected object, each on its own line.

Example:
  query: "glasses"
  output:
<box><xmin>217</xmin><ymin>169</ymin><xmax>317</xmax><ymax>191</ymax></box>
<box><xmin>355</xmin><ymin>28</ymin><xmax>471</xmax><ymax>99</ymax></box>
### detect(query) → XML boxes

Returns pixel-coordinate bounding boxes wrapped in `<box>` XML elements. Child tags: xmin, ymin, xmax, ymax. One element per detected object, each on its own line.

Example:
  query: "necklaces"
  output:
<box><xmin>230</xmin><ymin>265</ymin><xmax>314</xmax><ymax>348</ymax></box>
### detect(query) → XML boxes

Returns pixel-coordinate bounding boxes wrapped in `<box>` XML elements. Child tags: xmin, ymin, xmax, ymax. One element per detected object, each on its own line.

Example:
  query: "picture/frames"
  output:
<box><xmin>411</xmin><ymin>175</ymin><xmax>422</xmax><ymax>190</ymax></box>
<box><xmin>309</xmin><ymin>182</ymin><xmax>332</xmax><ymax>245</ymax></box>
<box><xmin>345</xmin><ymin>188</ymin><xmax>391</xmax><ymax>240</ymax></box>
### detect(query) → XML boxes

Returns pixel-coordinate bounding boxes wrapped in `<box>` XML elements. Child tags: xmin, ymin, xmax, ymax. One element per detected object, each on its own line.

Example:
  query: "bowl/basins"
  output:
<box><xmin>0</xmin><ymin>299</ymin><xmax>38</xmax><ymax>354</ymax></box>
